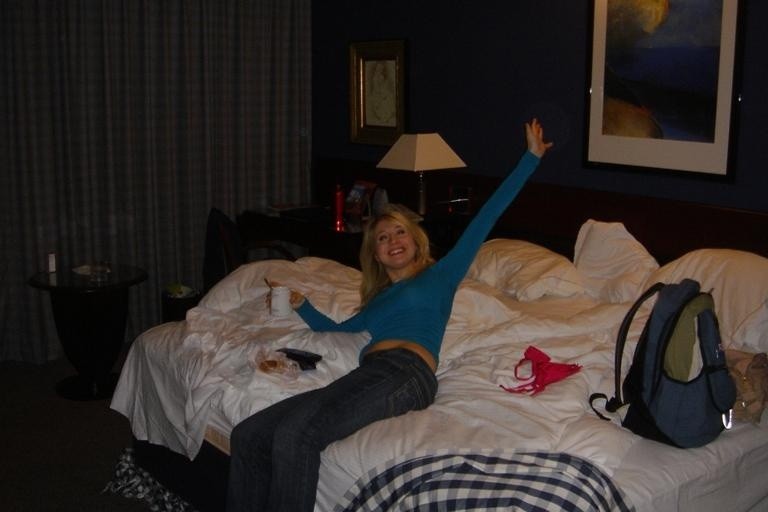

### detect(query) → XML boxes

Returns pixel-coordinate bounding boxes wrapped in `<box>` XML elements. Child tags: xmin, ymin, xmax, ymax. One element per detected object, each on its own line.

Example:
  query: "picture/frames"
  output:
<box><xmin>349</xmin><ymin>39</ymin><xmax>405</xmax><ymax>147</ymax></box>
<box><xmin>588</xmin><ymin>1</ymin><xmax>737</xmax><ymax>175</ymax></box>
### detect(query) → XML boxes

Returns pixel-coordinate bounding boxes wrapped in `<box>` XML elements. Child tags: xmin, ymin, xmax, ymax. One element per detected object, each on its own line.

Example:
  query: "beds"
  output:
<box><xmin>139</xmin><ymin>174</ymin><xmax>767</xmax><ymax>510</ymax></box>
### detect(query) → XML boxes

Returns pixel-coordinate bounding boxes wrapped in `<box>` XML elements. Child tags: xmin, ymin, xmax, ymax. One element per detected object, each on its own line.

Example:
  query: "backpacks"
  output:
<box><xmin>587</xmin><ymin>278</ymin><xmax>736</xmax><ymax>448</ymax></box>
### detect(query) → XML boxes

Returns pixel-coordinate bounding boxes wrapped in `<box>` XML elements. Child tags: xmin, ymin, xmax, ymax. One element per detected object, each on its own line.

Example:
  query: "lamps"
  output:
<box><xmin>375</xmin><ymin>132</ymin><xmax>467</xmax><ymax>216</ymax></box>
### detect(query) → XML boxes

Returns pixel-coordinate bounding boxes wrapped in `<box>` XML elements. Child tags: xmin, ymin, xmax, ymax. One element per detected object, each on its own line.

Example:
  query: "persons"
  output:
<box><xmin>223</xmin><ymin>117</ymin><xmax>554</xmax><ymax>511</ymax></box>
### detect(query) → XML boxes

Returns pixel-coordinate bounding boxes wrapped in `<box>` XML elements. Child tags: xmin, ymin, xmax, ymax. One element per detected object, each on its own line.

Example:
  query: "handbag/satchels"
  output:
<box><xmin>722</xmin><ymin>347</ymin><xmax>767</xmax><ymax>424</ymax></box>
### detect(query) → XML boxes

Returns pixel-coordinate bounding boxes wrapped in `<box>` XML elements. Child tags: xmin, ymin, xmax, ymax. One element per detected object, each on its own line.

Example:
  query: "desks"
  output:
<box><xmin>28</xmin><ymin>262</ymin><xmax>148</xmax><ymax>404</ymax></box>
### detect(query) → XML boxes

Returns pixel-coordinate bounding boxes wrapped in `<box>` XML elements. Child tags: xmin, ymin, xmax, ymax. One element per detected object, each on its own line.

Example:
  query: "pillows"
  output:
<box><xmin>643</xmin><ymin>247</ymin><xmax>768</xmax><ymax>354</ymax></box>
<box><xmin>465</xmin><ymin>238</ymin><xmax>575</xmax><ymax>302</ymax></box>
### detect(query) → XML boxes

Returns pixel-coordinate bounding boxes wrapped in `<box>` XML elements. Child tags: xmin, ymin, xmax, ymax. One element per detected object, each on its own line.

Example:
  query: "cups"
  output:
<box><xmin>271</xmin><ymin>285</ymin><xmax>293</xmax><ymax>319</ymax></box>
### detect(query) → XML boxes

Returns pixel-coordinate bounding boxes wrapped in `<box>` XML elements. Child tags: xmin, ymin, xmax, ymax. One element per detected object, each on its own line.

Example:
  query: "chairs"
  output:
<box><xmin>211</xmin><ymin>206</ymin><xmax>246</xmax><ymax>277</ymax></box>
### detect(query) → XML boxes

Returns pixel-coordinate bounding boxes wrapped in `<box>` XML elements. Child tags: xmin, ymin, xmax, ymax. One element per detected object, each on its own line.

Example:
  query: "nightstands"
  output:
<box><xmin>280</xmin><ymin>204</ymin><xmax>360</xmax><ymax>267</ymax></box>
